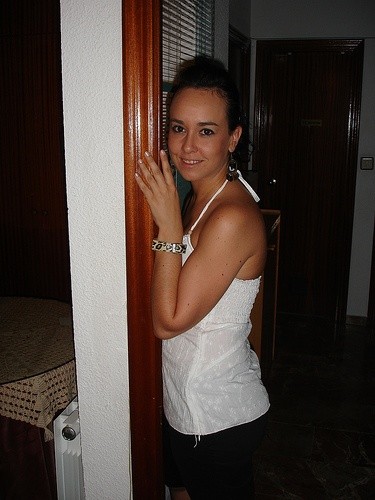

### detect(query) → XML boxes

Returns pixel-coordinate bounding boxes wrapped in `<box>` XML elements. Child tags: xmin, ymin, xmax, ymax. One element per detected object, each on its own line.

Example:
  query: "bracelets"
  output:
<box><xmin>149</xmin><ymin>238</ymin><xmax>187</xmax><ymax>256</ymax></box>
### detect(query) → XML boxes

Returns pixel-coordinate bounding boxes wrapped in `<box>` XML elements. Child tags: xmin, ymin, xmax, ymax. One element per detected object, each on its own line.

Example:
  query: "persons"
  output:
<box><xmin>133</xmin><ymin>54</ymin><xmax>275</xmax><ymax>500</ymax></box>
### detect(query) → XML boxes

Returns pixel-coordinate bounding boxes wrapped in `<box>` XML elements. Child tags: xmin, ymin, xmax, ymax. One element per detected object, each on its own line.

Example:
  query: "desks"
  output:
<box><xmin>0</xmin><ymin>295</ymin><xmax>78</xmax><ymax>500</ymax></box>
